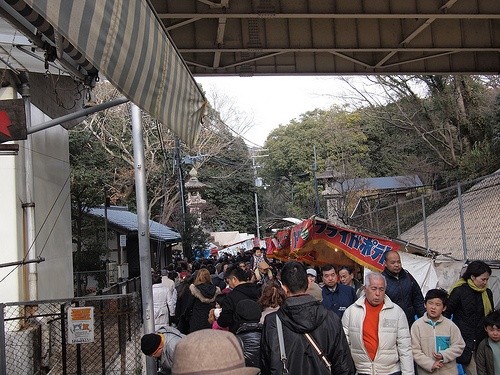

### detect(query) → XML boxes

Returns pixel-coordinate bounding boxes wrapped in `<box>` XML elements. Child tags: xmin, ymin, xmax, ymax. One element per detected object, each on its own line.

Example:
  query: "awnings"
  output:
<box><xmin>0</xmin><ymin>0</ymin><xmax>206</xmax><ymax>150</ymax></box>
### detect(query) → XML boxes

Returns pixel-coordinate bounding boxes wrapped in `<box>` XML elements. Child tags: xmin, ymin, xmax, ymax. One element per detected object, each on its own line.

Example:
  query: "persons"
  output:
<box><xmin>381</xmin><ymin>250</ymin><xmax>426</xmax><ymax>330</ymax></box>
<box><xmin>151</xmin><ymin>246</ymin><xmax>368</xmax><ymax>335</ymax></box>
<box><xmin>442</xmin><ymin>260</ymin><xmax>500</xmax><ymax>375</ymax></box>
<box><xmin>410</xmin><ymin>288</ymin><xmax>466</xmax><ymax>375</ymax></box>
<box><xmin>172</xmin><ymin>299</ymin><xmax>263</xmax><ymax>375</ymax></box>
<box><xmin>341</xmin><ymin>271</ymin><xmax>414</xmax><ymax>375</ymax></box>
<box><xmin>140</xmin><ymin>326</ymin><xmax>184</xmax><ymax>375</ymax></box>
<box><xmin>260</xmin><ymin>262</ymin><xmax>357</xmax><ymax>375</ymax></box>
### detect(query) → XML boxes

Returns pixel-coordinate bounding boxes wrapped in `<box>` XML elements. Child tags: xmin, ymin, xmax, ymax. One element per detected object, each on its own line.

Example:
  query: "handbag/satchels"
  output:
<box><xmin>455</xmin><ymin>349</ymin><xmax>472</xmax><ymax>365</ymax></box>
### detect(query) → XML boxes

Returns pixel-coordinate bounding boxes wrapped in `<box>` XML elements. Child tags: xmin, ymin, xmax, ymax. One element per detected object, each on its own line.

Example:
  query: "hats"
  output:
<box><xmin>306</xmin><ymin>268</ymin><xmax>317</xmax><ymax>277</ymax></box>
<box><xmin>140</xmin><ymin>333</ymin><xmax>161</xmax><ymax>356</ymax></box>
<box><xmin>258</xmin><ymin>262</ymin><xmax>270</xmax><ymax>270</ymax></box>
<box><xmin>232</xmin><ymin>299</ymin><xmax>262</xmax><ymax>324</ymax></box>
<box><xmin>171</xmin><ymin>329</ymin><xmax>261</xmax><ymax>375</ymax></box>
